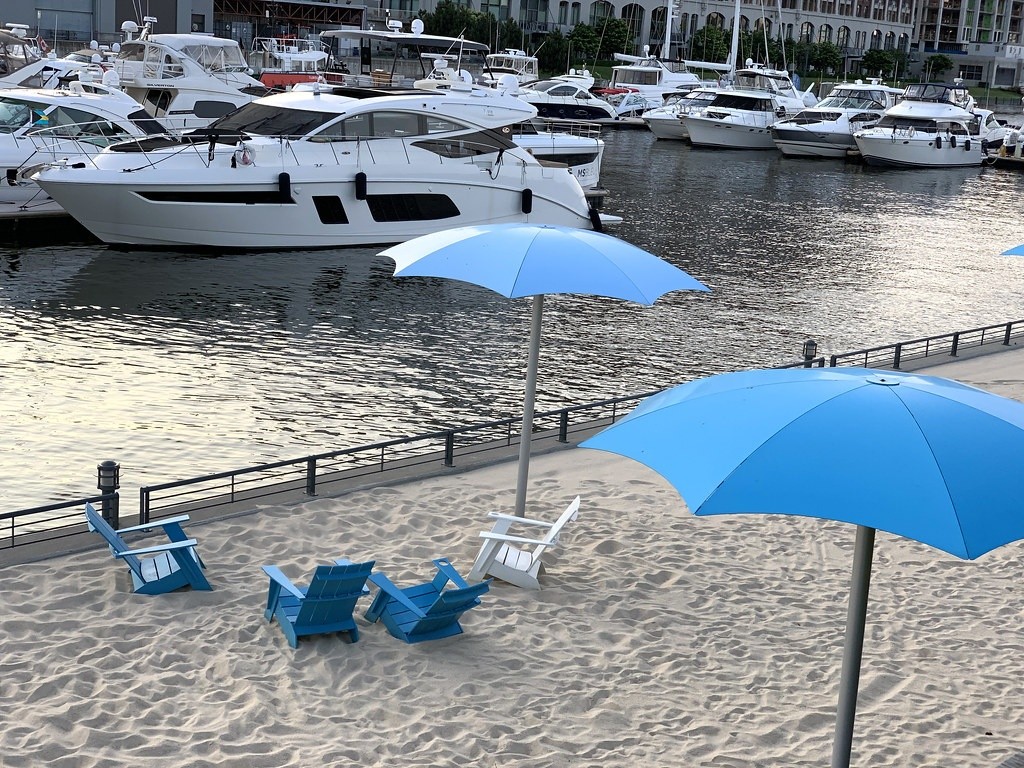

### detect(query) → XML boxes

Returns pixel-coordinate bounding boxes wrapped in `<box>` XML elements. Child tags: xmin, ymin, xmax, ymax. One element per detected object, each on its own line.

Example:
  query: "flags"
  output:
<box><xmin>36</xmin><ymin>34</ymin><xmax>50</xmax><ymax>52</ymax></box>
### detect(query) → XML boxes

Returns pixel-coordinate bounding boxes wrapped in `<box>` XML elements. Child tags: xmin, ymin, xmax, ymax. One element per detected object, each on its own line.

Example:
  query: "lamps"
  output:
<box><xmin>802</xmin><ymin>340</ymin><xmax>817</xmax><ymax>368</ymax></box>
<box><xmin>97</xmin><ymin>461</ymin><xmax>120</xmax><ymax>530</ymax></box>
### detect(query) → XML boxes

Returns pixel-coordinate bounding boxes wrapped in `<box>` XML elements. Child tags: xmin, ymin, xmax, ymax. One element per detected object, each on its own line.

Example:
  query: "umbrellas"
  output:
<box><xmin>999</xmin><ymin>244</ymin><xmax>1024</xmax><ymax>256</ymax></box>
<box><xmin>375</xmin><ymin>223</ymin><xmax>712</xmax><ymax>523</ymax></box>
<box><xmin>576</xmin><ymin>367</ymin><xmax>1024</xmax><ymax>768</ymax></box>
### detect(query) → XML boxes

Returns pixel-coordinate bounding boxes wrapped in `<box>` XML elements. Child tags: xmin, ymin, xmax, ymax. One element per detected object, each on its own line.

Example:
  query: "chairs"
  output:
<box><xmin>260</xmin><ymin>494</ymin><xmax>580</xmax><ymax>651</ymax></box>
<box><xmin>85</xmin><ymin>502</ymin><xmax>214</xmax><ymax>595</ymax></box>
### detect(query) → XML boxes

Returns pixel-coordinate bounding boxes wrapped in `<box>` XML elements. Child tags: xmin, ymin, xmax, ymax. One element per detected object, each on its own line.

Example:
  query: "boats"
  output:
<box><xmin>0</xmin><ymin>0</ymin><xmax>1024</xmax><ymax>253</ymax></box>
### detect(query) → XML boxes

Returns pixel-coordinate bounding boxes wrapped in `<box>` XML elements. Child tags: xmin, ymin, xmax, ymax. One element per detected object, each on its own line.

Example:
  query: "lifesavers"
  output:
<box><xmin>236</xmin><ymin>146</ymin><xmax>256</xmax><ymax>165</ymax></box>
